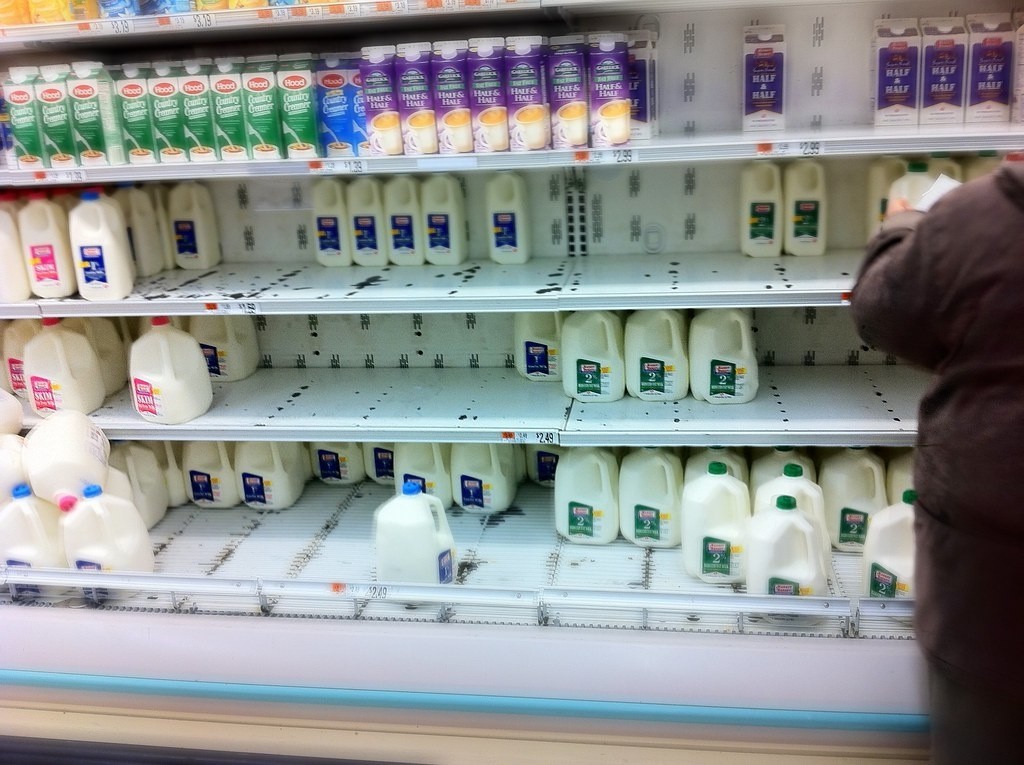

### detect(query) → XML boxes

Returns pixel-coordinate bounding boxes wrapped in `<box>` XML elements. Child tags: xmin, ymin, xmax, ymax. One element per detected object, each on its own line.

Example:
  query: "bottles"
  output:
<box><xmin>0</xmin><ymin>153</ymin><xmax>1024</xmax><ymax>598</ymax></box>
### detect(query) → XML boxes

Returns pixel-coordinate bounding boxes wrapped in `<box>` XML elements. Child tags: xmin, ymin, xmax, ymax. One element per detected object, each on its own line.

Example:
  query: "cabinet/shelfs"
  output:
<box><xmin>0</xmin><ymin>0</ymin><xmax>1024</xmax><ymax>764</ymax></box>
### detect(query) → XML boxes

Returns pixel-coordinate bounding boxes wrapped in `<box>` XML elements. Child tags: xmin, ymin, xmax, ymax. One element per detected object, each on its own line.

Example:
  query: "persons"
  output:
<box><xmin>843</xmin><ymin>158</ymin><xmax>1024</xmax><ymax>765</ymax></box>
